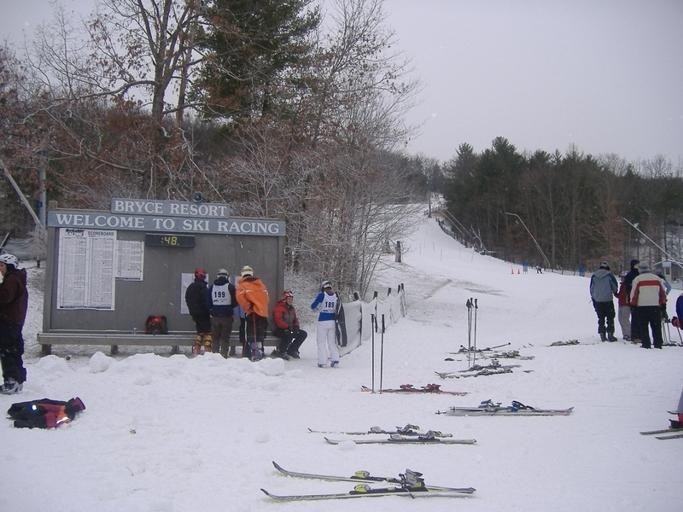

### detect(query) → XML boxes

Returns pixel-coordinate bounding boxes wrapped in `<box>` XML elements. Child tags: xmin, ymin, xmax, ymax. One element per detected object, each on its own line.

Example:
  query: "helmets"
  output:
<box><xmin>215</xmin><ymin>268</ymin><xmax>231</xmax><ymax>282</ymax></box>
<box><xmin>240</xmin><ymin>265</ymin><xmax>253</xmax><ymax>278</ymax></box>
<box><xmin>0</xmin><ymin>253</ymin><xmax>19</xmax><ymax>270</ymax></box>
<box><xmin>320</xmin><ymin>280</ymin><xmax>333</xmax><ymax>291</ymax></box>
<box><xmin>195</xmin><ymin>268</ymin><xmax>206</xmax><ymax>280</ymax></box>
<box><xmin>282</xmin><ymin>290</ymin><xmax>293</xmax><ymax>302</ymax></box>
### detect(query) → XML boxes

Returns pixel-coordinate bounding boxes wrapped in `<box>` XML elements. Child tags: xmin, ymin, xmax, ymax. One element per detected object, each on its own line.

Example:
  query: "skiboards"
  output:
<box><xmin>306</xmin><ymin>426</ymin><xmax>475</xmax><ymax>446</ymax></box>
<box><xmin>432</xmin><ymin>336</ymin><xmax>603</xmax><ymax>378</ymax></box>
<box><xmin>639</xmin><ymin>428</ymin><xmax>683</xmax><ymax>441</ymax></box>
<box><xmin>361</xmin><ymin>382</ymin><xmax>467</xmax><ymax>397</ymax></box>
<box><xmin>260</xmin><ymin>460</ymin><xmax>475</xmax><ymax>502</ymax></box>
<box><xmin>435</xmin><ymin>401</ymin><xmax>573</xmax><ymax>416</ymax></box>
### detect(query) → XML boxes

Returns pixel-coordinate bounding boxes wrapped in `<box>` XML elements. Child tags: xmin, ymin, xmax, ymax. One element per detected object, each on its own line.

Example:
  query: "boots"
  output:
<box><xmin>191</xmin><ymin>334</ymin><xmax>229</xmax><ymax>359</ymax></box>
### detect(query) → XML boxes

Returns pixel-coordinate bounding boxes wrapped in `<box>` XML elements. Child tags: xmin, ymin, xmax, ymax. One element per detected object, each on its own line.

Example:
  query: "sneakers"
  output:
<box><xmin>600</xmin><ymin>333</ymin><xmax>662</xmax><ymax>349</ymax></box>
<box><xmin>0</xmin><ymin>377</ymin><xmax>23</xmax><ymax>394</ymax></box>
<box><xmin>313</xmin><ymin>363</ymin><xmax>327</xmax><ymax>368</ymax></box>
<box><xmin>287</xmin><ymin>349</ymin><xmax>300</xmax><ymax>359</ymax></box>
<box><xmin>328</xmin><ymin>361</ymin><xmax>339</xmax><ymax>368</ymax></box>
<box><xmin>271</xmin><ymin>348</ymin><xmax>291</xmax><ymax>361</ymax></box>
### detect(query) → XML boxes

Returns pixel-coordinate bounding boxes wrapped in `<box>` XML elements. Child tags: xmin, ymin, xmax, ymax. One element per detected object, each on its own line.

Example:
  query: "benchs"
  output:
<box><xmin>35</xmin><ymin>331</ymin><xmax>289</xmax><ymax>360</ymax></box>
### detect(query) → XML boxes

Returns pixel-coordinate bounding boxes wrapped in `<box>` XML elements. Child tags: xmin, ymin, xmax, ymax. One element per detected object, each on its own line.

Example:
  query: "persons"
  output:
<box><xmin>536</xmin><ymin>266</ymin><xmax>542</xmax><ymax>274</ymax></box>
<box><xmin>588</xmin><ymin>259</ymin><xmax>682</xmax><ymax>349</ymax></box>
<box><xmin>183</xmin><ymin>264</ymin><xmax>342</xmax><ymax>370</ymax></box>
<box><xmin>0</xmin><ymin>250</ymin><xmax>29</xmax><ymax>395</ymax></box>
<box><xmin>666</xmin><ymin>387</ymin><xmax>682</xmax><ymax>427</ymax></box>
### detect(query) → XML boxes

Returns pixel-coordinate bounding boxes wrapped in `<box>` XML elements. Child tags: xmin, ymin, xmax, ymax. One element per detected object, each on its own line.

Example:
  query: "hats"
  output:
<box><xmin>631</xmin><ymin>260</ymin><xmax>654</xmax><ymax>273</ymax></box>
